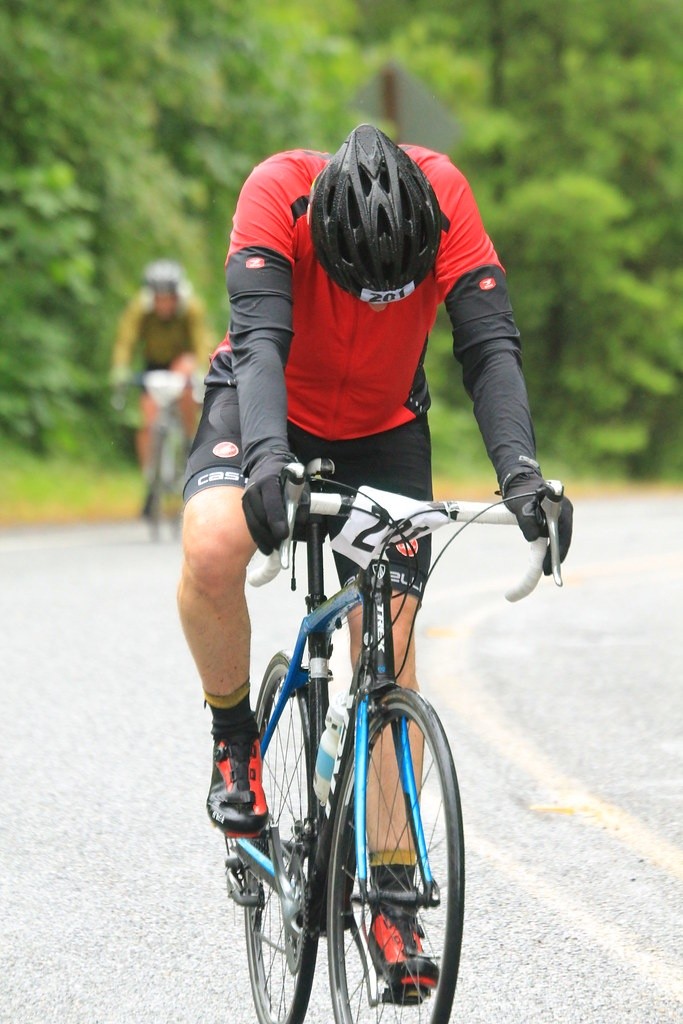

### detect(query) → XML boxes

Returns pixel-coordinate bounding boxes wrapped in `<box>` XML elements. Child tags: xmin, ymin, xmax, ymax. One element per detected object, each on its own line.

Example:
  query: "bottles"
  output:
<box><xmin>311</xmin><ymin>698</ymin><xmax>354</xmax><ymax>802</ymax></box>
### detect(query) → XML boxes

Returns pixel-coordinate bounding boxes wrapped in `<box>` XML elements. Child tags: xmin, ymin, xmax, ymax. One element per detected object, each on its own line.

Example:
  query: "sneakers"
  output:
<box><xmin>369</xmin><ymin>911</ymin><xmax>439</xmax><ymax>988</ymax></box>
<box><xmin>206</xmin><ymin>730</ymin><xmax>268</xmax><ymax>838</ymax></box>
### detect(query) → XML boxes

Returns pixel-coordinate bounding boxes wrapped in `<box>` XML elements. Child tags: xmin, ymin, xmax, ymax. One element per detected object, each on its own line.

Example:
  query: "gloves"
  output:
<box><xmin>241</xmin><ymin>444</ymin><xmax>305</xmax><ymax>556</ymax></box>
<box><xmin>503</xmin><ymin>472</ymin><xmax>573</xmax><ymax>577</ymax></box>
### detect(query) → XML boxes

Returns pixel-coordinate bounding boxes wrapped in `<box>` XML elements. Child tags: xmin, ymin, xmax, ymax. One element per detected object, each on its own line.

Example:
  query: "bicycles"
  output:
<box><xmin>127</xmin><ymin>370</ymin><xmax>207</xmax><ymax>545</ymax></box>
<box><xmin>222</xmin><ymin>458</ymin><xmax>563</xmax><ymax>1024</ymax></box>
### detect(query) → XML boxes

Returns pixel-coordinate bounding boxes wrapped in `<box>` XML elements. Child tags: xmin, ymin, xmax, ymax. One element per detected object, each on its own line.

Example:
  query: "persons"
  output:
<box><xmin>176</xmin><ymin>124</ymin><xmax>574</xmax><ymax>989</ymax></box>
<box><xmin>109</xmin><ymin>260</ymin><xmax>212</xmax><ymax>473</ymax></box>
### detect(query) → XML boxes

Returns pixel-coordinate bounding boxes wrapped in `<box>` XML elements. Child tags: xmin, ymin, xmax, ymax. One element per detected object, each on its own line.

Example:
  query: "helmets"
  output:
<box><xmin>146</xmin><ymin>261</ymin><xmax>179</xmax><ymax>290</ymax></box>
<box><xmin>307</xmin><ymin>126</ymin><xmax>451</xmax><ymax>303</ymax></box>
